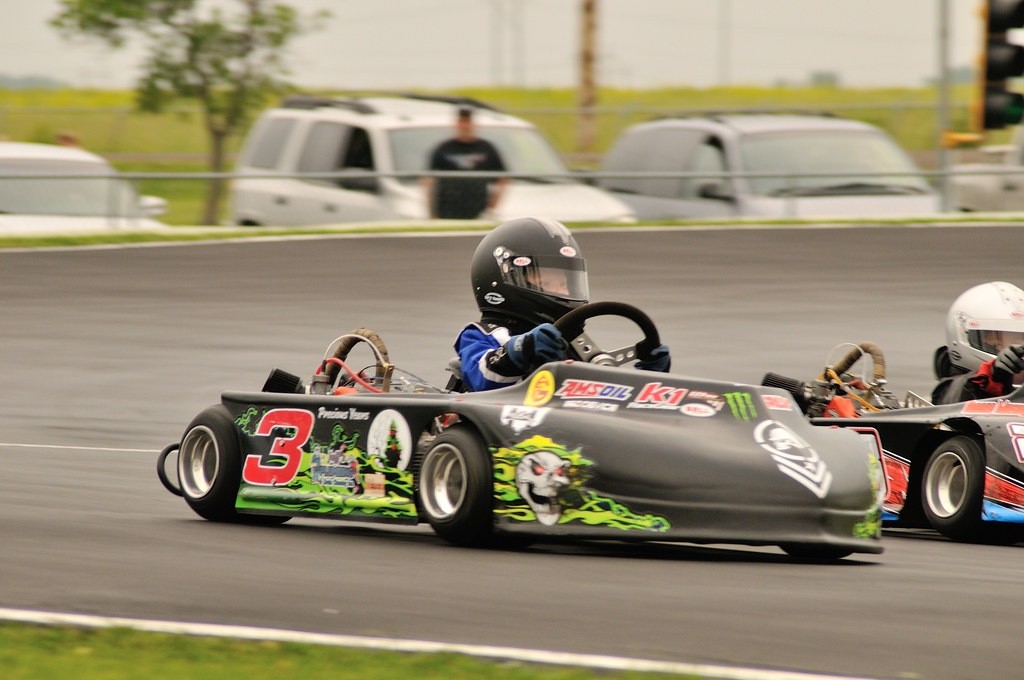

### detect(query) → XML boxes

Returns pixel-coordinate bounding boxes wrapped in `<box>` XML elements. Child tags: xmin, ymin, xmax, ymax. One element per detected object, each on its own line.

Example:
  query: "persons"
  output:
<box><xmin>931</xmin><ymin>280</ymin><xmax>1024</xmax><ymax>406</ymax></box>
<box><xmin>419</xmin><ymin>109</ymin><xmax>508</xmax><ymax>221</ymax></box>
<box><xmin>452</xmin><ymin>215</ymin><xmax>671</xmax><ymax>394</ymax></box>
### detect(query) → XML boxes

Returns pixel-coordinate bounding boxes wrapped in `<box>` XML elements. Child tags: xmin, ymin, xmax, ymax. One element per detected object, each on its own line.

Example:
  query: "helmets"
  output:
<box><xmin>945</xmin><ymin>281</ymin><xmax>1024</xmax><ymax>378</ymax></box>
<box><xmin>470</xmin><ymin>218</ymin><xmax>590</xmax><ymax>326</ymax></box>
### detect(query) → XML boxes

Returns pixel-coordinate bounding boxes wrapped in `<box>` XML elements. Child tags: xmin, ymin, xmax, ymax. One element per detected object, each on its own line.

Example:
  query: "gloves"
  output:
<box><xmin>508</xmin><ymin>322</ymin><xmax>568</xmax><ymax>367</ymax></box>
<box><xmin>994</xmin><ymin>344</ymin><xmax>1023</xmax><ymax>378</ymax></box>
<box><xmin>635</xmin><ymin>344</ymin><xmax>671</xmax><ymax>372</ymax></box>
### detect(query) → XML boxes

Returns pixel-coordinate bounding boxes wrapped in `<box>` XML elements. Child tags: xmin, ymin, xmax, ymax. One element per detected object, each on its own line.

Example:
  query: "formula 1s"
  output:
<box><xmin>158</xmin><ymin>301</ymin><xmax>889</xmax><ymax>566</ymax></box>
<box><xmin>761</xmin><ymin>342</ymin><xmax>1024</xmax><ymax>544</ymax></box>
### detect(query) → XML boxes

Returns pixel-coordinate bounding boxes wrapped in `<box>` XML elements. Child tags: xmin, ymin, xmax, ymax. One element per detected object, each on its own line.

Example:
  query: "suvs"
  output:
<box><xmin>229</xmin><ymin>94</ymin><xmax>638</xmax><ymax>224</ymax></box>
<box><xmin>596</xmin><ymin>113</ymin><xmax>951</xmax><ymax>221</ymax></box>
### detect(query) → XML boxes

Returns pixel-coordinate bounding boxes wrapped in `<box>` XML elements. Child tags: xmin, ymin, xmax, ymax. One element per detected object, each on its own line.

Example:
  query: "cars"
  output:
<box><xmin>0</xmin><ymin>141</ymin><xmax>167</xmax><ymax>231</ymax></box>
